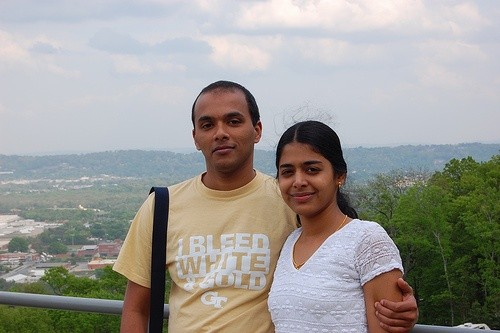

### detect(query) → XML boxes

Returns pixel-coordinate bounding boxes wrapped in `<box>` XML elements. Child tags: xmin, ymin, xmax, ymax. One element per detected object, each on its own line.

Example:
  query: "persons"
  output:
<box><xmin>112</xmin><ymin>80</ymin><xmax>418</xmax><ymax>333</ymax></box>
<box><xmin>268</xmin><ymin>121</ymin><xmax>404</xmax><ymax>333</ymax></box>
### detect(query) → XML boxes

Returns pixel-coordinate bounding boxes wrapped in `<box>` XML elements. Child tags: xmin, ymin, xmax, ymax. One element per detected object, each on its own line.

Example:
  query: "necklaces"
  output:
<box><xmin>292</xmin><ymin>215</ymin><xmax>347</xmax><ymax>269</ymax></box>
